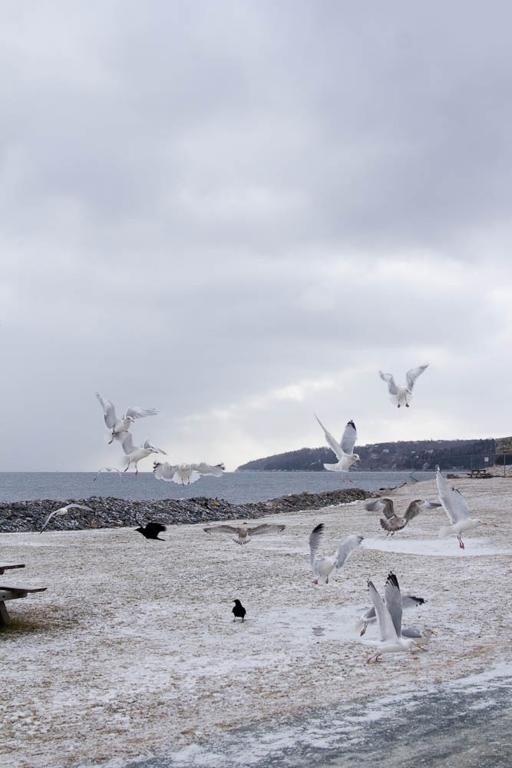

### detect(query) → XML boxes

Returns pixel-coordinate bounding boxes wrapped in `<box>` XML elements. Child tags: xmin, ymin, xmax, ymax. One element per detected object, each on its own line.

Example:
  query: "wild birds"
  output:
<box><xmin>313</xmin><ymin>411</ymin><xmax>361</xmax><ymax>483</ymax></box>
<box><xmin>153</xmin><ymin>462</ymin><xmax>225</xmax><ymax>487</ymax></box>
<box><xmin>364</xmin><ymin>467</ymin><xmax>488</xmax><ymax>550</ymax></box>
<box><xmin>379</xmin><ymin>364</ymin><xmax>430</xmax><ymax>408</ymax></box>
<box><xmin>232</xmin><ymin>599</ymin><xmax>245</xmax><ymax>623</ymax></box>
<box><xmin>360</xmin><ymin>572</ymin><xmax>437</xmax><ymax>667</ymax></box>
<box><xmin>309</xmin><ymin>523</ymin><xmax>364</xmax><ymax>584</ymax></box>
<box><xmin>204</xmin><ymin>524</ymin><xmax>285</xmax><ymax>544</ymax></box>
<box><xmin>93</xmin><ymin>393</ymin><xmax>167</xmax><ymax>483</ymax></box>
<box><xmin>134</xmin><ymin>520</ymin><xmax>166</xmax><ymax>541</ymax></box>
<box><xmin>37</xmin><ymin>504</ymin><xmax>94</xmax><ymax>535</ymax></box>
